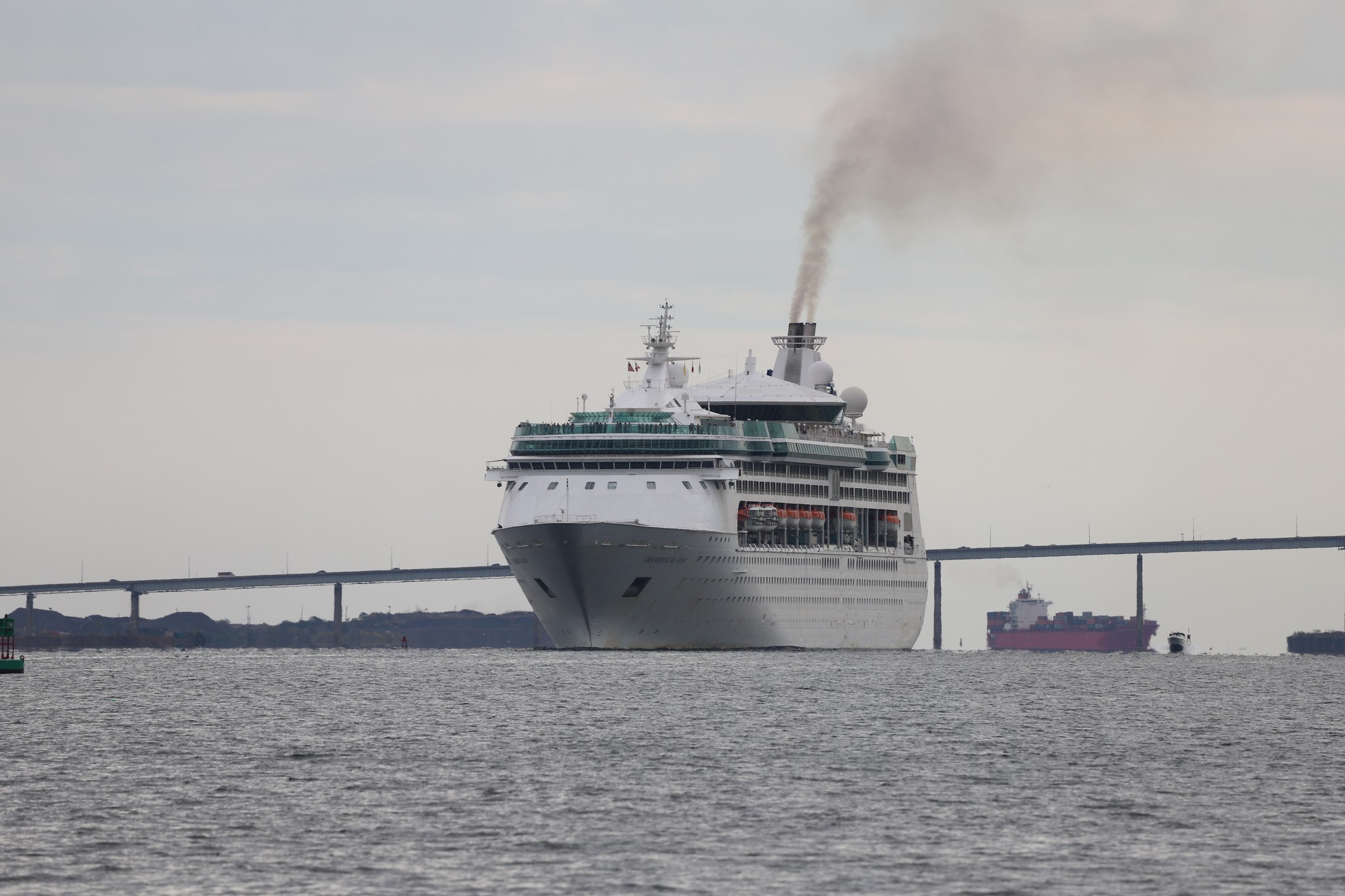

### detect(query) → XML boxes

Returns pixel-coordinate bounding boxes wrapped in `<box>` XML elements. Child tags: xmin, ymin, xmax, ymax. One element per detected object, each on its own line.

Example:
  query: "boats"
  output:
<box><xmin>798</xmin><ymin>511</ymin><xmax>813</xmax><ymax>525</ymax></box>
<box><xmin>876</xmin><ymin>514</ymin><xmax>900</xmax><ymax>531</ymax></box>
<box><xmin>987</xmin><ymin>580</ymin><xmax>1159</xmax><ymax>653</ymax></box>
<box><xmin>777</xmin><ymin>508</ymin><xmax>788</xmax><ymax>525</ymax></box>
<box><xmin>788</xmin><ymin>509</ymin><xmax>799</xmax><ymax>526</ymax></box>
<box><xmin>1287</xmin><ymin>630</ymin><xmax>1345</xmax><ymax>658</ymax></box>
<box><xmin>832</xmin><ymin>511</ymin><xmax>856</xmax><ymax>530</ymax></box>
<box><xmin>812</xmin><ymin>511</ymin><xmax>826</xmax><ymax>527</ymax></box>
<box><xmin>737</xmin><ymin>503</ymin><xmax>779</xmax><ymax>531</ymax></box>
<box><xmin>1168</xmin><ymin>631</ymin><xmax>1190</xmax><ymax>655</ymax></box>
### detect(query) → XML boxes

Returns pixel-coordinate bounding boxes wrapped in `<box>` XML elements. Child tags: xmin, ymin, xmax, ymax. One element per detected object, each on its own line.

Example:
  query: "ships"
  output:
<box><xmin>483</xmin><ymin>300</ymin><xmax>927</xmax><ymax>653</ymax></box>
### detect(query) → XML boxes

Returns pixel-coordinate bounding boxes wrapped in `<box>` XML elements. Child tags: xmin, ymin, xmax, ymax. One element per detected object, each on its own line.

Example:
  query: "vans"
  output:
<box><xmin>218</xmin><ymin>572</ymin><xmax>235</xmax><ymax>577</ymax></box>
<box><xmin>490</xmin><ymin>564</ymin><xmax>499</xmax><ymax>566</ymax></box>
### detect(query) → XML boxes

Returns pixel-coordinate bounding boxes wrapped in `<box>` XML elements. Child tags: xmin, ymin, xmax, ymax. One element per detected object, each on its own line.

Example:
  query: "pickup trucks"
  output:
<box><xmin>958</xmin><ymin>546</ymin><xmax>970</xmax><ymax>549</ymax></box>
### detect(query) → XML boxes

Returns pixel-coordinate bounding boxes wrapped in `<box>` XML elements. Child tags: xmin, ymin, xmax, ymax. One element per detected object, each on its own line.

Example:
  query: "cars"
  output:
<box><xmin>109</xmin><ymin>579</ymin><xmax>119</xmax><ymax>581</ymax></box>
<box><xmin>390</xmin><ymin>568</ymin><xmax>401</xmax><ymax>570</ymax></box>
<box><xmin>316</xmin><ymin>570</ymin><xmax>326</xmax><ymax>573</ymax></box>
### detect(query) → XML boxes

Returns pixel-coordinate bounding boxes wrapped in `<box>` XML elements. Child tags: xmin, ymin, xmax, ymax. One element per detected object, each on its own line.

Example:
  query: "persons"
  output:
<box><xmin>518</xmin><ymin>422</ymin><xmax>575</xmax><ymax>436</ymax></box>
<box><xmin>582</xmin><ymin>422</ymin><xmax>607</xmax><ymax>434</ymax></box>
<box><xmin>638</xmin><ymin>422</ymin><xmax>678</xmax><ymax>434</ymax></box>
<box><xmin>616</xmin><ymin>421</ymin><xmax>631</xmax><ymax>433</ymax></box>
<box><xmin>802</xmin><ymin>430</ymin><xmax>807</xmax><ymax>434</ymax></box>
<box><xmin>689</xmin><ymin>423</ymin><xmax>719</xmax><ymax>435</ymax></box>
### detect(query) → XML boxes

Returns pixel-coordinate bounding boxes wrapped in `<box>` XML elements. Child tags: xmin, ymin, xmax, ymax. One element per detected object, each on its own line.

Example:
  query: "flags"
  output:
<box><xmin>684</xmin><ymin>361</ymin><xmax>686</xmax><ymax>376</ymax></box>
<box><xmin>692</xmin><ymin>360</ymin><xmax>694</xmax><ymax>373</ymax></box>
<box><xmin>628</xmin><ymin>361</ymin><xmax>635</xmax><ymax>372</ymax></box>
<box><xmin>635</xmin><ymin>361</ymin><xmax>640</xmax><ymax>372</ymax></box>
<box><xmin>699</xmin><ymin>360</ymin><xmax>701</xmax><ymax>374</ymax></box>
